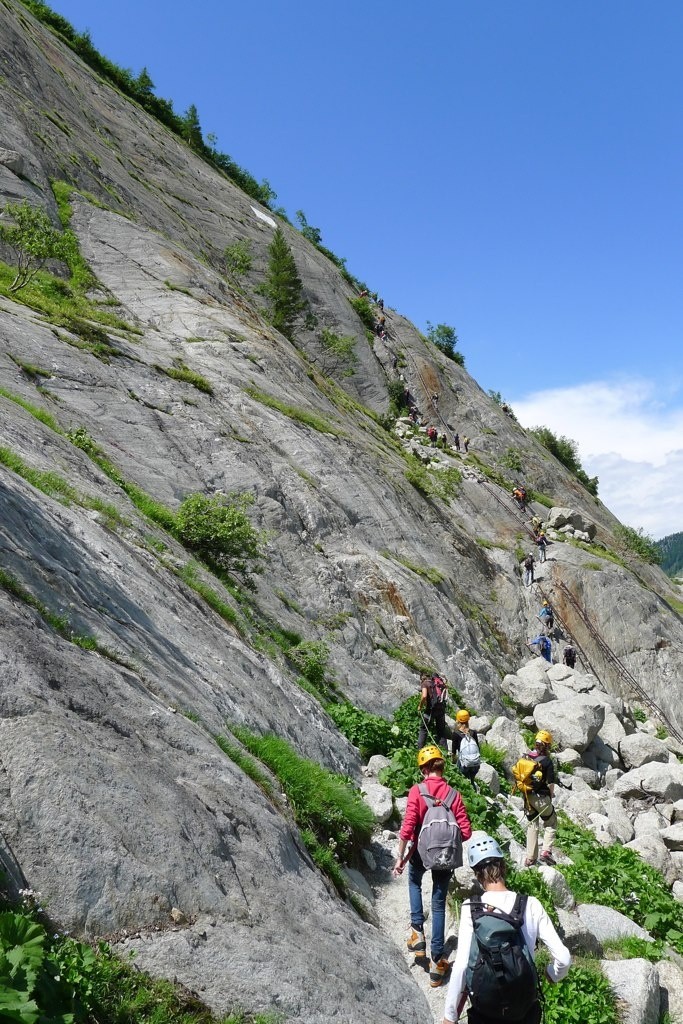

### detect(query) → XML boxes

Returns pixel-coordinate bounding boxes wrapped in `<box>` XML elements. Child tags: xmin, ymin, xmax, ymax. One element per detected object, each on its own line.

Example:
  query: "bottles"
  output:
<box><xmin>535</xmin><ymin>771</ymin><xmax>542</xmax><ymax>780</ymax></box>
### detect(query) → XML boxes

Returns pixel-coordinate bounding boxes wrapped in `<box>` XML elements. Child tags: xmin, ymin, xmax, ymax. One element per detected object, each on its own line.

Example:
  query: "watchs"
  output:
<box><xmin>396</xmin><ymin>851</ymin><xmax>403</xmax><ymax>857</ymax></box>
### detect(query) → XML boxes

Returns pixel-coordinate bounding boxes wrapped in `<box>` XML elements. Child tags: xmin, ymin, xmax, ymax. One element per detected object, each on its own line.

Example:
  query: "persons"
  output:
<box><xmin>563</xmin><ymin>639</ymin><xmax>576</xmax><ymax>669</ymax></box>
<box><xmin>536</xmin><ymin>532</ymin><xmax>547</xmax><ymax>563</ymax></box>
<box><xmin>443</xmin><ymin>835</ymin><xmax>571</xmax><ymax>1024</ymax></box>
<box><xmin>392</xmin><ymin>746</ymin><xmax>471</xmax><ymax>989</ymax></box>
<box><xmin>361</xmin><ymin>289</ymin><xmax>384</xmax><ymax>311</ymax></box>
<box><xmin>409</xmin><ymin>406</ymin><xmax>427</xmax><ymax>427</ymax></box>
<box><xmin>452</xmin><ymin>710</ymin><xmax>480</xmax><ymax>792</ymax></box>
<box><xmin>511</xmin><ymin>487</ymin><xmax>527</xmax><ymax>513</ymax></box>
<box><xmin>525</xmin><ymin>633</ymin><xmax>552</xmax><ymax>663</ymax></box>
<box><xmin>428</xmin><ymin>427</ymin><xmax>447</xmax><ymax>449</ymax></box>
<box><xmin>375</xmin><ymin>316</ymin><xmax>387</xmax><ymax>346</ymax></box>
<box><xmin>454</xmin><ymin>433</ymin><xmax>470</xmax><ymax>452</ymax></box>
<box><xmin>417</xmin><ymin>673</ymin><xmax>448</xmax><ymax>750</ymax></box>
<box><xmin>431</xmin><ymin>392</ymin><xmax>440</xmax><ymax>410</ymax></box>
<box><xmin>523</xmin><ymin>513</ymin><xmax>542</xmax><ymax>538</ymax></box>
<box><xmin>535</xmin><ymin>601</ymin><xmax>554</xmax><ymax>633</ymax></box>
<box><xmin>515</xmin><ymin>729</ymin><xmax>559</xmax><ymax>866</ymax></box>
<box><xmin>524</xmin><ymin>552</ymin><xmax>536</xmax><ymax>587</ymax></box>
<box><xmin>391</xmin><ymin>355</ymin><xmax>397</xmax><ymax>369</ymax></box>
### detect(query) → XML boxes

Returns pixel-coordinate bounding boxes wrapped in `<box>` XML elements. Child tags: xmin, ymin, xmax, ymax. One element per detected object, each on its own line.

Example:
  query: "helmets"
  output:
<box><xmin>534</xmin><ymin>514</ymin><xmax>540</xmax><ymax>516</ymax></box>
<box><xmin>543</xmin><ymin>601</ymin><xmax>548</xmax><ymax>606</ymax></box>
<box><xmin>455</xmin><ymin>709</ymin><xmax>470</xmax><ymax>723</ymax></box>
<box><xmin>540</xmin><ymin>531</ymin><xmax>544</xmax><ymax>536</ymax></box>
<box><xmin>467</xmin><ymin>836</ymin><xmax>505</xmax><ymax>868</ymax></box>
<box><xmin>512</xmin><ymin>487</ymin><xmax>517</xmax><ymax>492</ymax></box>
<box><xmin>417</xmin><ymin>745</ymin><xmax>443</xmax><ymax>766</ymax></box>
<box><xmin>535</xmin><ymin>730</ymin><xmax>552</xmax><ymax>745</ymax></box>
<box><xmin>539</xmin><ymin>633</ymin><xmax>545</xmax><ymax>636</ymax></box>
<box><xmin>529</xmin><ymin>552</ymin><xmax>534</xmax><ymax>556</ymax></box>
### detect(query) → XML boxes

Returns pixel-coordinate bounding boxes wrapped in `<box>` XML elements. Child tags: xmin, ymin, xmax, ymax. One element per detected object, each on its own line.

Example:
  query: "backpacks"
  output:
<box><xmin>417</xmin><ymin>782</ymin><xmax>463</xmax><ymax>870</ymax></box>
<box><xmin>453</xmin><ymin>729</ymin><xmax>482</xmax><ymax>767</ymax></box>
<box><xmin>524</xmin><ymin>557</ymin><xmax>532</xmax><ymax>570</ymax></box>
<box><xmin>536</xmin><ymin>536</ymin><xmax>544</xmax><ymax>545</ymax></box>
<box><xmin>512</xmin><ymin>753</ymin><xmax>549</xmax><ymax>811</ymax></box>
<box><xmin>461</xmin><ymin>892</ymin><xmax>545</xmax><ymax>1024</ymax></box>
<box><xmin>540</xmin><ymin>638</ymin><xmax>548</xmax><ymax>652</ymax></box>
<box><xmin>563</xmin><ymin>646</ymin><xmax>574</xmax><ymax>659</ymax></box>
<box><xmin>422</xmin><ymin>673</ymin><xmax>449</xmax><ymax>709</ymax></box>
<box><xmin>544</xmin><ymin>606</ymin><xmax>552</xmax><ymax>616</ymax></box>
<box><xmin>517</xmin><ymin>488</ymin><xmax>527</xmax><ymax>495</ymax></box>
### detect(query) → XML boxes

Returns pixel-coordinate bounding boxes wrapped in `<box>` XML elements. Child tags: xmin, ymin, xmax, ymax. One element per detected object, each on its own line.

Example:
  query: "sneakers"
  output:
<box><xmin>427</xmin><ymin>951</ymin><xmax>449</xmax><ymax>988</ymax></box>
<box><xmin>523</xmin><ymin>858</ymin><xmax>541</xmax><ymax>867</ymax></box>
<box><xmin>406</xmin><ymin>924</ymin><xmax>426</xmax><ymax>957</ymax></box>
<box><xmin>540</xmin><ymin>850</ymin><xmax>556</xmax><ymax>866</ymax></box>
